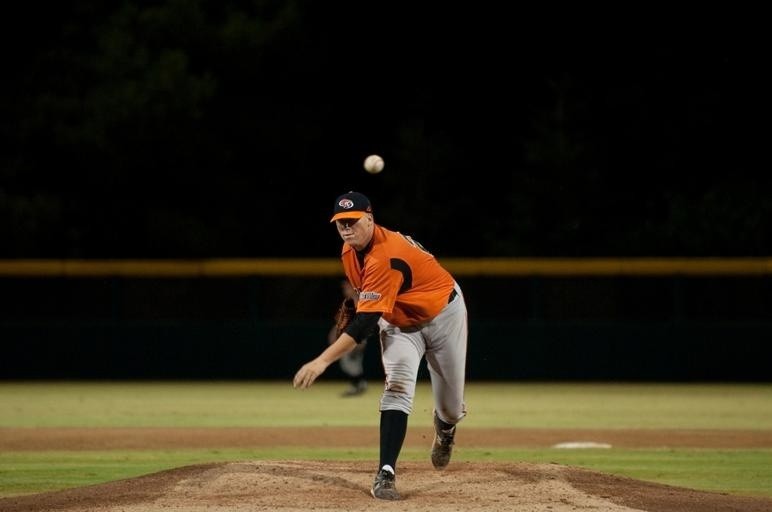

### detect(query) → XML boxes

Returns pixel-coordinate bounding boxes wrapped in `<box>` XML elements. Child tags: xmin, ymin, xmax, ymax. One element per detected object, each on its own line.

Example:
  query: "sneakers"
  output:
<box><xmin>370</xmin><ymin>465</ymin><xmax>399</xmax><ymax>500</ymax></box>
<box><xmin>431</xmin><ymin>408</ymin><xmax>456</xmax><ymax>470</ymax></box>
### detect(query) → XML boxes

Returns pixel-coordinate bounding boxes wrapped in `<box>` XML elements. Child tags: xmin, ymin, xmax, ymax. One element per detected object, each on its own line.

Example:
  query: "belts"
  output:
<box><xmin>448</xmin><ymin>289</ymin><xmax>457</xmax><ymax>304</ymax></box>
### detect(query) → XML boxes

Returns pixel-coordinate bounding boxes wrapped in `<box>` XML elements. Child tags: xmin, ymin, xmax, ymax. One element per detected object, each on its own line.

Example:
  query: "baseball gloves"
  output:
<box><xmin>336</xmin><ymin>296</ymin><xmax>357</xmax><ymax>341</ymax></box>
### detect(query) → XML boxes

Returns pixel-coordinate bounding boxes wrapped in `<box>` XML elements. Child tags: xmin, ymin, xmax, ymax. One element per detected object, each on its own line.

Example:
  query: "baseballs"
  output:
<box><xmin>364</xmin><ymin>154</ymin><xmax>384</xmax><ymax>175</ymax></box>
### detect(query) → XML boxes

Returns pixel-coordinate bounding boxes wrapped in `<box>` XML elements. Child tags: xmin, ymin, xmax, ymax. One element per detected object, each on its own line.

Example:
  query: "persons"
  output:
<box><xmin>328</xmin><ymin>280</ymin><xmax>368</xmax><ymax>399</ymax></box>
<box><xmin>293</xmin><ymin>188</ymin><xmax>470</xmax><ymax>502</ymax></box>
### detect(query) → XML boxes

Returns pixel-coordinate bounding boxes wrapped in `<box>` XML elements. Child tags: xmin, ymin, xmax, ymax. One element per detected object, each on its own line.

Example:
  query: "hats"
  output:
<box><xmin>330</xmin><ymin>191</ymin><xmax>370</xmax><ymax>223</ymax></box>
